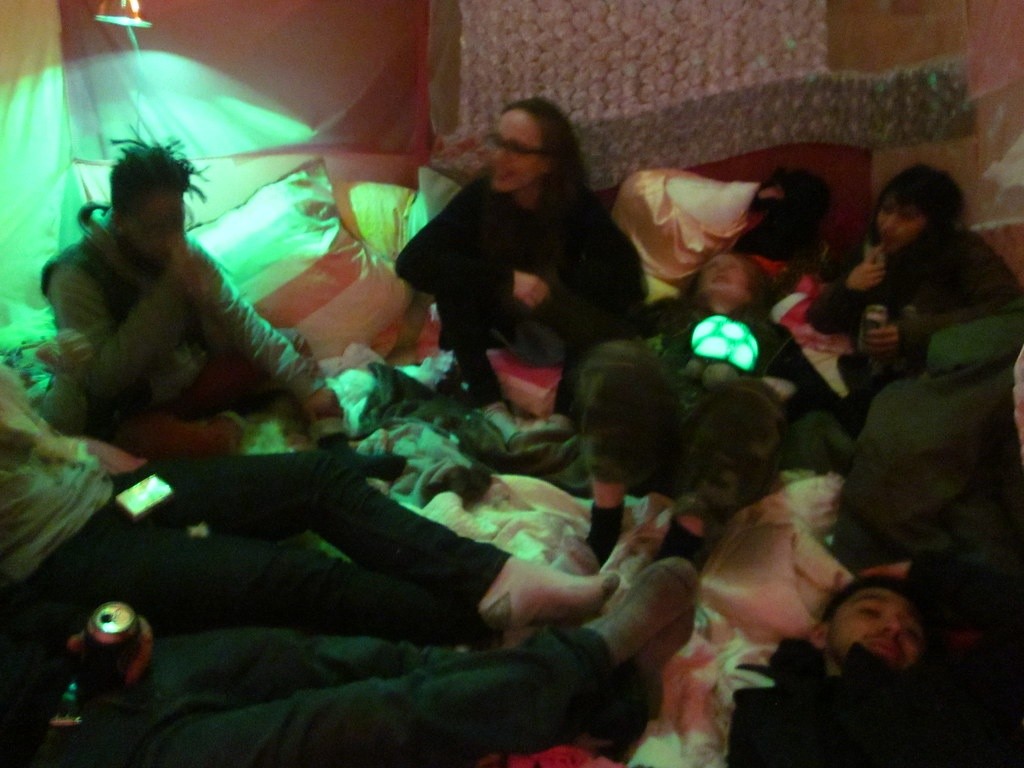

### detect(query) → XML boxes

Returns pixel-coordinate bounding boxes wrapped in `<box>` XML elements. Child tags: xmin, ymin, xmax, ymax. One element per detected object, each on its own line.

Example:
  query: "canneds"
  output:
<box><xmin>859</xmin><ymin>304</ymin><xmax>889</xmax><ymax>353</ymax></box>
<box><xmin>84</xmin><ymin>601</ymin><xmax>140</xmax><ymax>690</ymax></box>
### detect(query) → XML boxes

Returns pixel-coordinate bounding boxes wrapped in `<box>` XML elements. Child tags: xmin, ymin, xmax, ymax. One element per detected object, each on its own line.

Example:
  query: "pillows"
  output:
<box><xmin>185</xmin><ymin>157</ymin><xmax>414</xmax><ymax>361</ymax></box>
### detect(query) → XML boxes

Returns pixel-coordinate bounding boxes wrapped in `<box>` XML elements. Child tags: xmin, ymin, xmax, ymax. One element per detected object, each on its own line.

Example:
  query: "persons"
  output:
<box><xmin>393</xmin><ymin>94</ymin><xmax>649</xmax><ymax>417</ymax></box>
<box><xmin>577</xmin><ymin>165</ymin><xmax>1024</xmax><ymax>768</ymax></box>
<box><xmin>1</xmin><ymin>360</ymin><xmax>622</xmax><ymax>649</ymax></box>
<box><xmin>0</xmin><ymin>555</ymin><xmax>699</xmax><ymax>767</ymax></box>
<box><xmin>38</xmin><ymin>139</ymin><xmax>408</xmax><ymax>480</ymax></box>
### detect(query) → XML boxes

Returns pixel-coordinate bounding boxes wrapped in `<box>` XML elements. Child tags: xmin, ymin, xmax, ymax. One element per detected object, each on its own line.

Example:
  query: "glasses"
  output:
<box><xmin>485</xmin><ymin>132</ymin><xmax>547</xmax><ymax>156</ymax></box>
